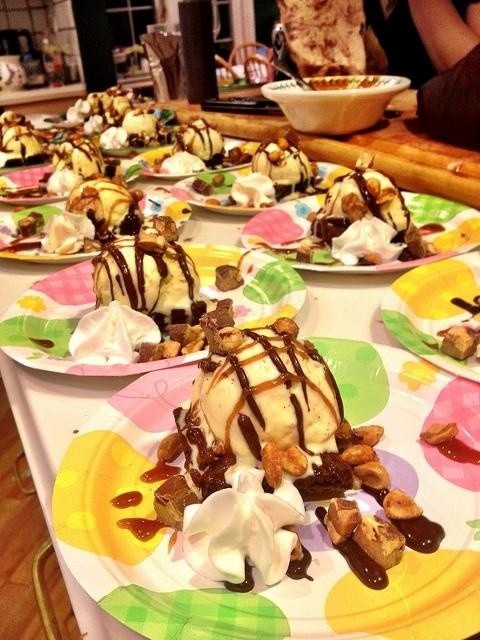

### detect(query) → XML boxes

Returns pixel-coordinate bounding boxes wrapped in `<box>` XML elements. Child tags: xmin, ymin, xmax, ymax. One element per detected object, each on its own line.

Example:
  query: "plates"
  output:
<box><xmin>133</xmin><ymin>133</ymin><xmax>263</xmax><ymax>179</ymax></box>
<box><xmin>1</xmin><ymin>183</ymin><xmax>191</xmax><ymax>264</ymax></box>
<box><xmin>237</xmin><ymin>185</ymin><xmax>480</xmax><ymax>276</ymax></box>
<box><xmin>379</xmin><ymin>254</ymin><xmax>479</xmax><ymax>383</ymax></box>
<box><xmin>1</xmin><ymin>240</ymin><xmax>308</xmax><ymax>379</ymax></box>
<box><xmin>0</xmin><ymin>155</ymin><xmax>143</xmax><ymax>205</ymax></box>
<box><xmin>51</xmin><ymin>336</ymin><xmax>478</xmax><ymax>629</ymax></box>
<box><xmin>170</xmin><ymin>158</ymin><xmax>351</xmax><ymax>216</ymax></box>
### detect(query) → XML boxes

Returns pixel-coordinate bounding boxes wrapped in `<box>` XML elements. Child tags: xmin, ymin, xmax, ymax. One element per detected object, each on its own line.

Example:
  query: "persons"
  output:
<box><xmin>364</xmin><ymin>0</ymin><xmax>480</xmax><ymax>87</ymax></box>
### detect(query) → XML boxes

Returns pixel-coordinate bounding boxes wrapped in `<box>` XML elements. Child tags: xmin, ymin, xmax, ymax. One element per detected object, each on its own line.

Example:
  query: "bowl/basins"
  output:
<box><xmin>261</xmin><ymin>69</ymin><xmax>412</xmax><ymax>137</ymax></box>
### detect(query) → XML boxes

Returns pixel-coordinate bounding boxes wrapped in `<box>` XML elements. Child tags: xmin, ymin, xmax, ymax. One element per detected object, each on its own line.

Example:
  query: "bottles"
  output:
<box><xmin>40</xmin><ymin>27</ymin><xmax>64</xmax><ymax>86</ymax></box>
<box><xmin>18</xmin><ymin>37</ymin><xmax>45</xmax><ymax>93</ymax></box>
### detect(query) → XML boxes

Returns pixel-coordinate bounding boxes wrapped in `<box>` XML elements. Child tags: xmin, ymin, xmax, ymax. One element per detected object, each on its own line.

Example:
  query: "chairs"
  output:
<box><xmin>217</xmin><ymin>41</ymin><xmax>275</xmax><ymax>99</ymax></box>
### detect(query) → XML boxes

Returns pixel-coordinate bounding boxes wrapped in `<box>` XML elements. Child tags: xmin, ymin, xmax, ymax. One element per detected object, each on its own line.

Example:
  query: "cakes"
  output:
<box><xmin>86</xmin><ymin>89</ymin><xmax>132</xmax><ymax>128</ymax></box>
<box><xmin>3</xmin><ymin>124</ymin><xmax>42</xmax><ymax>160</ymax></box>
<box><xmin>65</xmin><ymin>178</ymin><xmax>144</xmax><ymax>233</ymax></box>
<box><xmin>120</xmin><ymin>106</ymin><xmax>159</xmax><ymax>148</ymax></box>
<box><xmin>89</xmin><ymin>212</ymin><xmax>204</xmax><ymax>335</ymax></box>
<box><xmin>0</xmin><ymin>112</ymin><xmax>27</xmax><ymax>147</ymax></box>
<box><xmin>171</xmin><ymin>116</ymin><xmax>226</xmax><ymax>168</ymax></box>
<box><xmin>52</xmin><ymin>133</ymin><xmax>104</xmax><ymax>185</ymax></box>
<box><xmin>186</xmin><ymin>313</ymin><xmax>354</xmax><ymax>497</ymax></box>
<box><xmin>253</xmin><ymin>137</ymin><xmax>317</xmax><ymax>200</ymax></box>
<box><xmin>309</xmin><ymin>150</ymin><xmax>425</xmax><ymax>262</ymax></box>
